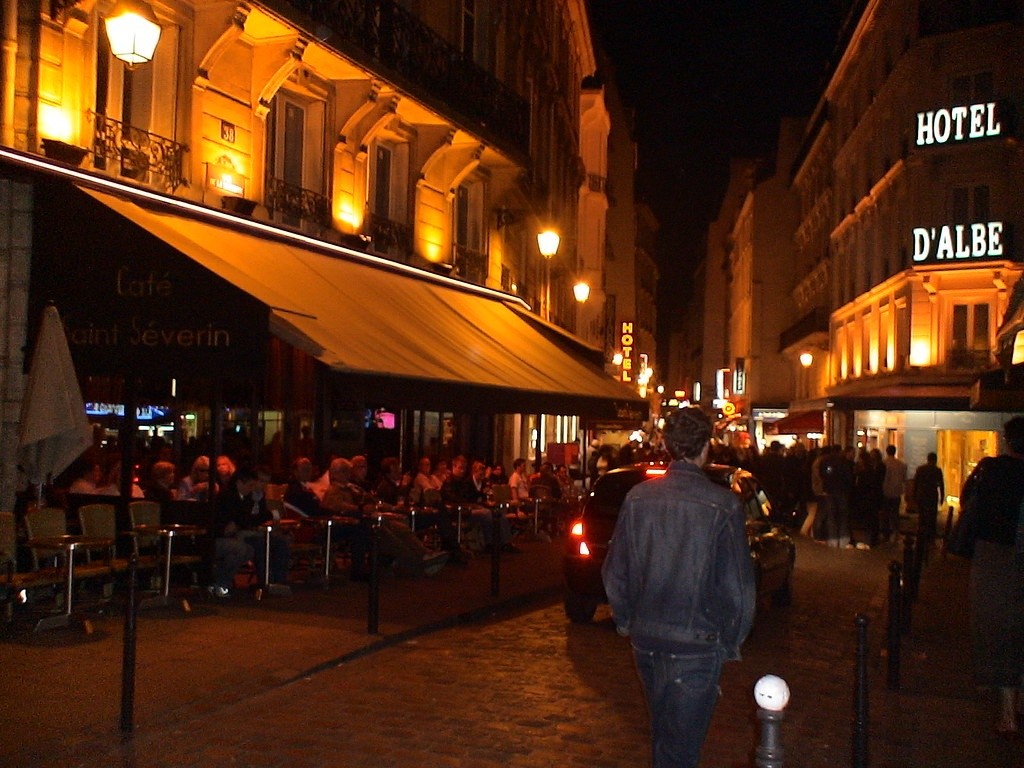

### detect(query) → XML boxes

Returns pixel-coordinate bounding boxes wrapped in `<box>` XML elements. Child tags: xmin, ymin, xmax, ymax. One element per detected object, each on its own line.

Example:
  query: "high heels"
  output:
<box><xmin>1015</xmin><ymin>713</ymin><xmax>1024</xmax><ymax>728</ymax></box>
<box><xmin>993</xmin><ymin>724</ymin><xmax>1017</xmax><ymax>742</ymax></box>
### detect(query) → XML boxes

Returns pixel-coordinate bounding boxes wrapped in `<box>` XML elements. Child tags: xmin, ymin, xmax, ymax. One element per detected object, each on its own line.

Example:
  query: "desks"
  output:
<box><xmin>25</xmin><ymin>495</ymin><xmax>586</xmax><ymax>633</ymax></box>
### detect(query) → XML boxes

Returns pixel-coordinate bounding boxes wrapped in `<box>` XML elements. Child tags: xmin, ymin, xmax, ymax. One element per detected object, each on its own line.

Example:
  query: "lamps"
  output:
<box><xmin>222</xmin><ymin>195</ymin><xmax>259</xmax><ymax>214</ymax></box>
<box><xmin>751</xmin><ymin>671</ymin><xmax>793</xmax><ymax>711</ymax></box>
<box><xmin>433</xmin><ymin>261</ymin><xmax>455</xmax><ymax>274</ymax></box>
<box><xmin>495</xmin><ymin>206</ymin><xmax>561</xmax><ymax>259</ymax></box>
<box><xmin>39</xmin><ymin>137</ymin><xmax>91</xmax><ymax>167</ymax></box>
<box><xmin>550</xmin><ymin>266</ymin><xmax>590</xmax><ymax>303</ymax></box>
<box><xmin>341</xmin><ymin>233</ymin><xmax>371</xmax><ymax>249</ymax></box>
<box><xmin>49</xmin><ymin>0</ymin><xmax>165</xmax><ymax>66</ymax></box>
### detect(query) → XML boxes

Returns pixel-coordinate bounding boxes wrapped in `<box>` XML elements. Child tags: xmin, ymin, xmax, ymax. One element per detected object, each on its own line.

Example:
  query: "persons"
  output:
<box><xmin>75</xmin><ymin>407</ymin><xmax>1024</xmax><ymax>738</ymax></box>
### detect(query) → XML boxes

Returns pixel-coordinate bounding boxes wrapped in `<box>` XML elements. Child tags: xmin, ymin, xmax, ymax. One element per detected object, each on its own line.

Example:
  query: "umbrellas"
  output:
<box><xmin>21</xmin><ymin>302</ymin><xmax>92</xmax><ymax>505</ymax></box>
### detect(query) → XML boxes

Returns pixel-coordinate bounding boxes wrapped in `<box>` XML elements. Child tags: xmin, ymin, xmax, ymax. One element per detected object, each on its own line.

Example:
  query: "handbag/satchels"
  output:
<box><xmin>947</xmin><ymin>457</ymin><xmax>992</xmax><ymax>560</ymax></box>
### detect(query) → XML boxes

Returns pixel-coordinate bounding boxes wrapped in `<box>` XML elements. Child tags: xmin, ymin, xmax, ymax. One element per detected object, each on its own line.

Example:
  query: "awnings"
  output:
<box><xmin>762</xmin><ymin>411</ymin><xmax>828</xmax><ymax>435</ymax></box>
<box><xmin>71</xmin><ymin>180</ymin><xmax>650</xmax><ymax>423</ymax></box>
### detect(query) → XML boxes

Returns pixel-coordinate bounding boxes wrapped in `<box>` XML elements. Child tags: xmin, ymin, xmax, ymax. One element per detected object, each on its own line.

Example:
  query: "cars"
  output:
<box><xmin>564</xmin><ymin>459</ymin><xmax>796</xmax><ymax>633</ymax></box>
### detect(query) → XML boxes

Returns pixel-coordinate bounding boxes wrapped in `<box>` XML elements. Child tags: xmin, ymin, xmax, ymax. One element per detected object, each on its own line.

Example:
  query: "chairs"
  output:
<box><xmin>24</xmin><ymin>507</ymin><xmax>110</xmax><ymax>610</ymax></box>
<box><xmin>491</xmin><ymin>485</ymin><xmax>535</xmax><ymax>542</ymax></box>
<box><xmin>125</xmin><ymin>500</ymin><xmax>199</xmax><ymax>595</ymax></box>
<box><xmin>0</xmin><ymin>512</ymin><xmax>42</xmax><ymax>630</ymax></box>
<box><xmin>78</xmin><ymin>502</ymin><xmax>150</xmax><ymax>597</ymax></box>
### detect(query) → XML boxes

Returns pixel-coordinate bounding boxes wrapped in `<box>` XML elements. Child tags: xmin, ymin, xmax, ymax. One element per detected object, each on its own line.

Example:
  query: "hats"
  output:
<box><xmin>771</xmin><ymin>441</ymin><xmax>784</xmax><ymax>447</ymax></box>
